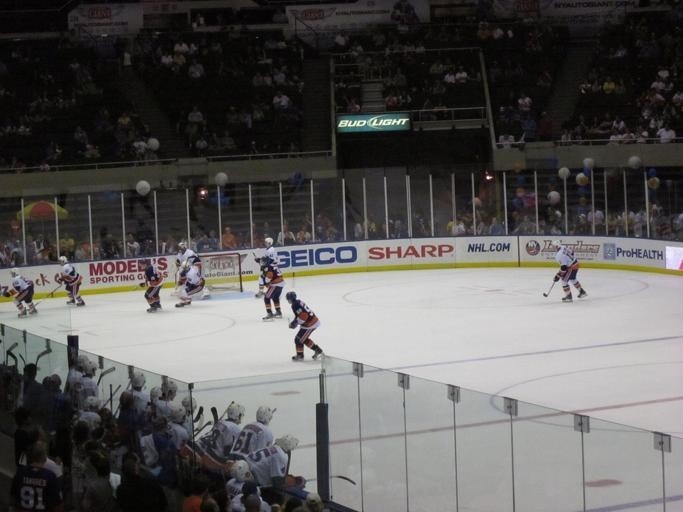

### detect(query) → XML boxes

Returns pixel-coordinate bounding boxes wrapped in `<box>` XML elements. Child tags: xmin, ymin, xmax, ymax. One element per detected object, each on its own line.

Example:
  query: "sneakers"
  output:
<box><xmin>562</xmin><ymin>295</ymin><xmax>572</xmax><ymax>300</ymax></box>
<box><xmin>293</xmin><ymin>353</ymin><xmax>305</xmax><ymax>360</ymax></box>
<box><xmin>312</xmin><ymin>346</ymin><xmax>324</xmax><ymax>360</ymax></box>
<box><xmin>578</xmin><ymin>288</ymin><xmax>586</xmax><ymax>297</ymax></box>
<box><xmin>18</xmin><ymin>289</ymin><xmax>283</xmax><ymax>320</ymax></box>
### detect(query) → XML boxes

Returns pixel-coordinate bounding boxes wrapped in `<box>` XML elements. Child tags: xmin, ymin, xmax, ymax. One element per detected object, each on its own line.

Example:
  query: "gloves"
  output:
<box><xmin>288</xmin><ymin>319</ymin><xmax>298</xmax><ymax>329</ymax></box>
<box><xmin>553</xmin><ymin>276</ymin><xmax>560</xmax><ymax>282</ymax></box>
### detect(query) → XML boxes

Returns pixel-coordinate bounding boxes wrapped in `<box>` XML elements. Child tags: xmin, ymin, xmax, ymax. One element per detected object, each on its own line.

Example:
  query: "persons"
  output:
<box><xmin>10</xmin><ymin>272</ymin><xmax>36</xmax><ymax>318</ymax></box>
<box><xmin>53</xmin><ymin>255</ymin><xmax>84</xmax><ymax>306</ymax></box>
<box><xmin>284</xmin><ymin>293</ymin><xmax>322</xmax><ymax>360</ymax></box>
<box><xmin>553</xmin><ymin>242</ymin><xmax>586</xmax><ymax>304</ymax></box>
<box><xmin>1</xmin><ymin>14</ymin><xmax>681</xmax><ymax>267</ymax></box>
<box><xmin>137</xmin><ymin>259</ymin><xmax>162</xmax><ymax>312</ymax></box>
<box><xmin>257</xmin><ymin>238</ymin><xmax>274</xmax><ymax>297</ymax></box>
<box><xmin>175</xmin><ymin>260</ymin><xmax>204</xmax><ymax>307</ymax></box>
<box><xmin>257</xmin><ymin>261</ymin><xmax>282</xmax><ymax>318</ymax></box>
<box><xmin>1</xmin><ymin>348</ymin><xmax>330</xmax><ymax>512</ymax></box>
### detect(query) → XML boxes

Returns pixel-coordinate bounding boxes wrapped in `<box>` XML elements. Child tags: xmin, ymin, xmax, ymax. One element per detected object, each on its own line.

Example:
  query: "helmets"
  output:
<box><xmin>76</xmin><ymin>354</ymin><xmax>101</xmax><ymax>409</ymax></box>
<box><xmin>227</xmin><ymin>402</ymin><xmax>246</xmax><ymax>424</ymax></box>
<box><xmin>230</xmin><ymin>459</ymin><xmax>253</xmax><ymax>482</ymax></box>
<box><xmin>551</xmin><ymin>240</ymin><xmax>562</xmax><ymax>249</ymax></box>
<box><xmin>286</xmin><ymin>291</ymin><xmax>298</xmax><ymax>304</ymax></box>
<box><xmin>257</xmin><ymin>405</ymin><xmax>273</xmax><ymax>425</ymax></box>
<box><xmin>12</xmin><ymin>237</ymin><xmax>277</xmax><ymax>277</ymax></box>
<box><xmin>130</xmin><ymin>370</ymin><xmax>146</xmax><ymax>391</ymax></box>
<box><xmin>150</xmin><ymin>378</ymin><xmax>197</xmax><ymax>423</ymax></box>
<box><xmin>278</xmin><ymin>434</ymin><xmax>299</xmax><ymax>454</ymax></box>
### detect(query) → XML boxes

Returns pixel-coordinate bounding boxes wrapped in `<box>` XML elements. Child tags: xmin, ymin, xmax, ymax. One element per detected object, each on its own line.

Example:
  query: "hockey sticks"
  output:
<box><xmin>36</xmin><ymin>348</ymin><xmax>52</xmax><ymax>366</ymax></box>
<box><xmin>95</xmin><ymin>366</ymin><xmax>115</xmax><ymax>386</ymax></box>
<box><xmin>543</xmin><ymin>281</ymin><xmax>555</xmax><ymax>297</ymax></box>
<box><xmin>6</xmin><ymin>342</ymin><xmax>18</xmax><ymax>368</ymax></box>
<box><xmin>306</xmin><ymin>475</ymin><xmax>356</xmax><ymax>486</ymax></box>
<box><xmin>193</xmin><ymin>406</ymin><xmax>218</xmax><ymax>438</ymax></box>
<box><xmin>26</xmin><ymin>285</ymin><xmax>63</xmax><ymax>311</ymax></box>
